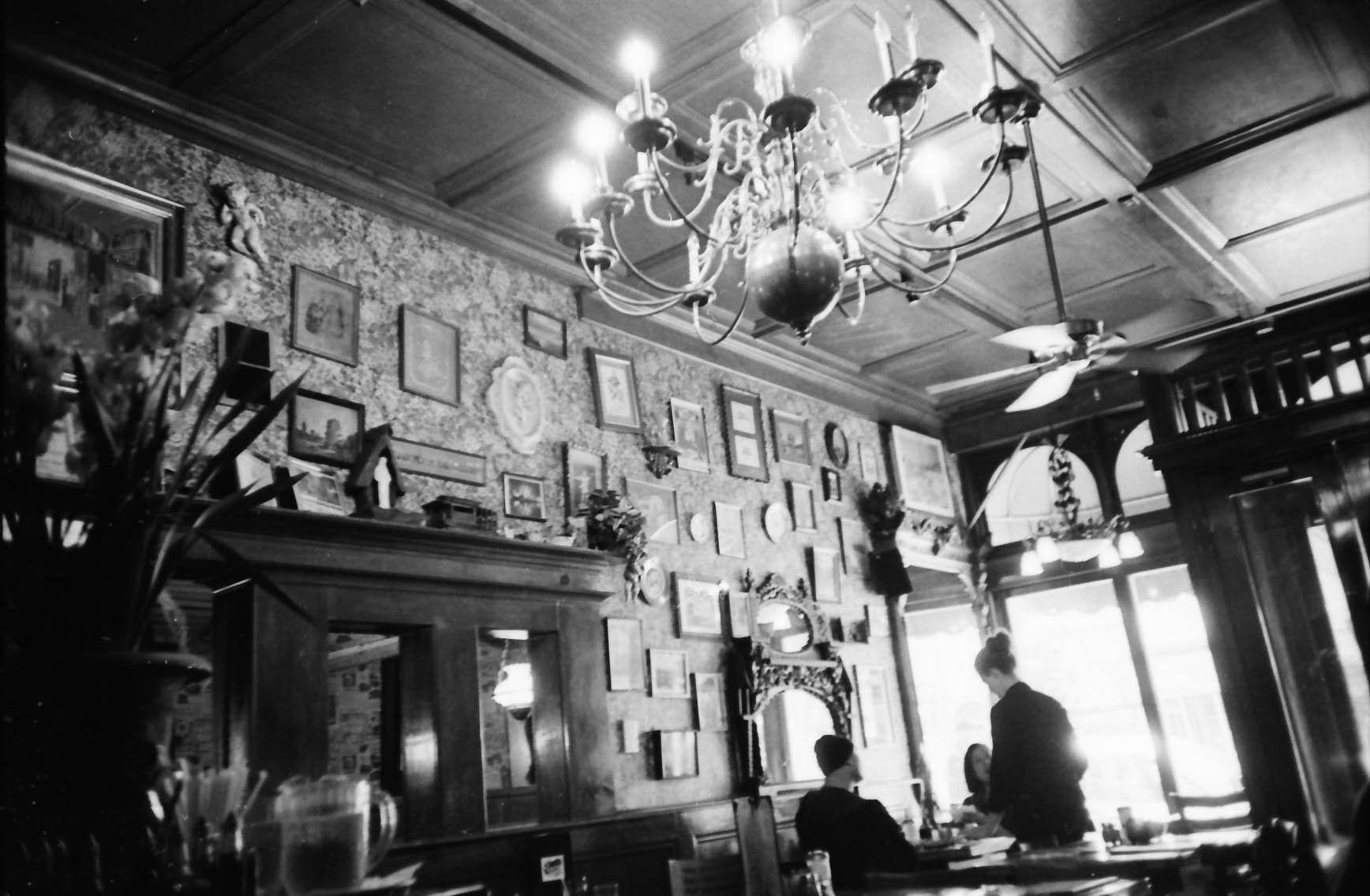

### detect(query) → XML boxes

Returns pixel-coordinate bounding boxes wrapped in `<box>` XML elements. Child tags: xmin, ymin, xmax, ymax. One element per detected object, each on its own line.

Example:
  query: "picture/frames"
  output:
<box><xmin>216</xmin><ymin>264</ymin><xmax>956</xmax><ymax>779</ymax></box>
<box><xmin>4</xmin><ymin>140</ymin><xmax>188</xmax><ymax>417</ymax></box>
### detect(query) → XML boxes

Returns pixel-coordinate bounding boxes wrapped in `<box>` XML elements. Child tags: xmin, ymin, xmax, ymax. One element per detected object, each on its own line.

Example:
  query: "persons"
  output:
<box><xmin>963</xmin><ymin>743</ymin><xmax>998</xmax><ymax>815</ymax></box>
<box><xmin>794</xmin><ymin>734</ymin><xmax>930</xmax><ymax>892</ymax></box>
<box><xmin>962</xmin><ymin>631</ymin><xmax>1097</xmax><ymax>852</ymax></box>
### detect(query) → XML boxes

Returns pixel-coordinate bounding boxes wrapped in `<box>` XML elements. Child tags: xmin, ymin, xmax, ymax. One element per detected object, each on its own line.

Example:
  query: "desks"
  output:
<box><xmin>859</xmin><ymin>820</ymin><xmax>1297</xmax><ymax>895</ymax></box>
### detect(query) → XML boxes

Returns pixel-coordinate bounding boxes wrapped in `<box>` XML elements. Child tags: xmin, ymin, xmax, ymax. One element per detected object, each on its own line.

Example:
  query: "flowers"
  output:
<box><xmin>2</xmin><ymin>254</ymin><xmax>320</xmax><ymax>666</ymax></box>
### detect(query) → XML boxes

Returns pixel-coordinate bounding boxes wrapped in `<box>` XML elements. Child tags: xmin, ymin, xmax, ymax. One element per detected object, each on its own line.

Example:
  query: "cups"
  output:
<box><xmin>274</xmin><ymin>780</ymin><xmax>399</xmax><ymax>894</ymax></box>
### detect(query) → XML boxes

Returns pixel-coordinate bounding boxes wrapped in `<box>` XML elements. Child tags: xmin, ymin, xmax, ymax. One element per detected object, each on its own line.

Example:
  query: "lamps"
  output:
<box><xmin>547</xmin><ymin>1</ymin><xmax>1034</xmax><ymax>347</ymax></box>
<box><xmin>1016</xmin><ymin>350</ymin><xmax>1145</xmax><ymax>575</ymax></box>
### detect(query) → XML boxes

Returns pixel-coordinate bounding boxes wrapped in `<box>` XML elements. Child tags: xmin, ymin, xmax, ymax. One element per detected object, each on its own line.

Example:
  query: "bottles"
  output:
<box><xmin>901</xmin><ymin>806</ymin><xmax>932</xmax><ymax>841</ymax></box>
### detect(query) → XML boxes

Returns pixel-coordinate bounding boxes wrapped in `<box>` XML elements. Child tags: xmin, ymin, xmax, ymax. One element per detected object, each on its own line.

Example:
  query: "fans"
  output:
<box><xmin>924</xmin><ymin>95</ymin><xmax>1216</xmax><ymax>415</ymax></box>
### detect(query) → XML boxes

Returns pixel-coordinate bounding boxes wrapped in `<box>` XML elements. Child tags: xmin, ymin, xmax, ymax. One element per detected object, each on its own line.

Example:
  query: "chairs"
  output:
<box><xmin>1169</xmin><ymin>777</ymin><xmax>1263</xmax><ymax>833</ymax></box>
<box><xmin>667</xmin><ymin>853</ymin><xmax>744</xmax><ymax>896</ymax></box>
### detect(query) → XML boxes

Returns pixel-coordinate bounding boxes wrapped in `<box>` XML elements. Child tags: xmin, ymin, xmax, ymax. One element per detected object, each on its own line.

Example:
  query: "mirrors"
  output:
<box><xmin>736</xmin><ymin>567</ymin><xmax>863</xmax><ymax>794</ymax></box>
